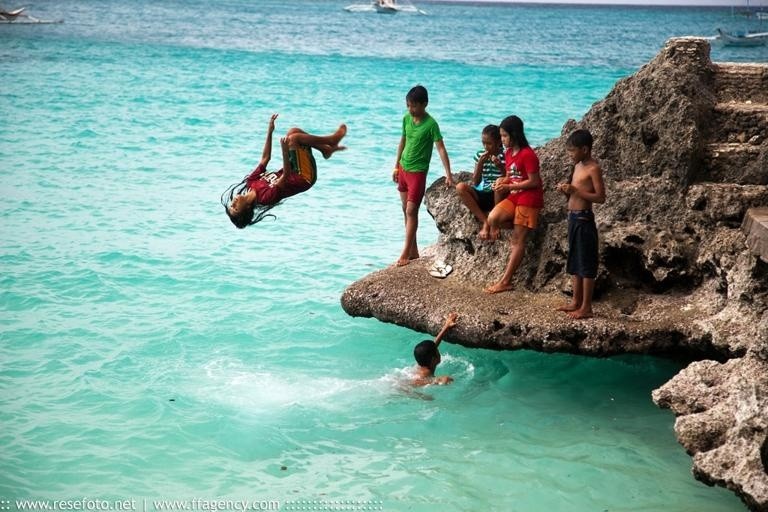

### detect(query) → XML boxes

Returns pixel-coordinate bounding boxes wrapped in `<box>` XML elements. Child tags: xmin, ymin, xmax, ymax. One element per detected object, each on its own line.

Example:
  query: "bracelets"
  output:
<box><xmin>392</xmin><ymin>169</ymin><xmax>399</xmax><ymax>173</ymax></box>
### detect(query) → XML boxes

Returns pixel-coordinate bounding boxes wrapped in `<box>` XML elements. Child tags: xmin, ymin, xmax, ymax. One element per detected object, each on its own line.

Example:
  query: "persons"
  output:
<box><xmin>221</xmin><ymin>114</ymin><xmax>347</xmax><ymax>229</ymax></box>
<box><xmin>392</xmin><ymin>83</ymin><xmax>452</xmax><ymax>266</ymax></box>
<box><xmin>456</xmin><ymin>116</ymin><xmax>544</xmax><ymax>294</ymax></box>
<box><xmin>556</xmin><ymin>130</ymin><xmax>605</xmax><ymax>319</ymax></box>
<box><xmin>398</xmin><ymin>312</ymin><xmax>458</xmax><ymax>401</ymax></box>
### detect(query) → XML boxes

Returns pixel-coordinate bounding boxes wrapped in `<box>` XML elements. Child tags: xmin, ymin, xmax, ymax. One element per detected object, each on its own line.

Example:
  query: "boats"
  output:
<box><xmin>0</xmin><ymin>8</ymin><xmax>63</xmax><ymax>23</ymax></box>
<box><xmin>717</xmin><ymin>27</ymin><xmax>768</xmax><ymax>47</ymax></box>
<box><xmin>344</xmin><ymin>3</ymin><xmax>418</xmax><ymax>12</ymax></box>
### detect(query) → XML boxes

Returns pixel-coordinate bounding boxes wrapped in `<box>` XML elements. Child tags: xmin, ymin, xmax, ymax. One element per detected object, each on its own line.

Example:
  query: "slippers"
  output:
<box><xmin>429</xmin><ymin>260</ymin><xmax>454</xmax><ymax>278</ymax></box>
